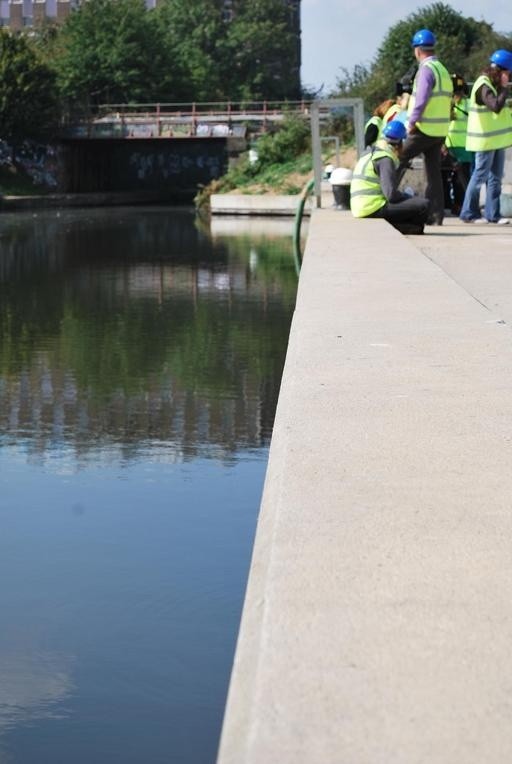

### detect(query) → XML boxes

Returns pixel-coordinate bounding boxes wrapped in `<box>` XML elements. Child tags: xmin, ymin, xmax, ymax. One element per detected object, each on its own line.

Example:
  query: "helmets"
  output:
<box><xmin>382</xmin><ymin>121</ymin><xmax>405</xmax><ymax>139</ymax></box>
<box><xmin>412</xmin><ymin>30</ymin><xmax>439</xmax><ymax>46</ymax></box>
<box><xmin>488</xmin><ymin>50</ymin><xmax>512</xmax><ymax>69</ymax></box>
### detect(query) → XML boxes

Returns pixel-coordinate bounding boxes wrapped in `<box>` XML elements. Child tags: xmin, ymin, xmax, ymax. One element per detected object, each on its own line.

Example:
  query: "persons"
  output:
<box><xmin>382</xmin><ymin>29</ymin><xmax>481</xmax><ymax>226</ymax></box>
<box><xmin>459</xmin><ymin>49</ymin><xmax>512</xmax><ymax>226</ymax></box>
<box><xmin>362</xmin><ymin>99</ymin><xmax>395</xmax><ymax>146</ymax></box>
<box><xmin>349</xmin><ymin>120</ymin><xmax>431</xmax><ymax>234</ymax></box>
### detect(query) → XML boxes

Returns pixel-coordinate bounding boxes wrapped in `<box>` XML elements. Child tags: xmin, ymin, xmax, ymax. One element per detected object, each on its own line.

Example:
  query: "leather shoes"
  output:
<box><xmin>426</xmin><ymin>209</ymin><xmax>445</xmax><ymax>225</ymax></box>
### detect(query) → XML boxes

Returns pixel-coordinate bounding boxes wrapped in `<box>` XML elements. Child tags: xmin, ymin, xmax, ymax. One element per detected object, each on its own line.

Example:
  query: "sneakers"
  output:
<box><xmin>497</xmin><ymin>222</ymin><xmax>510</xmax><ymax>224</ymax></box>
<box><xmin>463</xmin><ymin>217</ymin><xmax>488</xmax><ymax>224</ymax></box>
